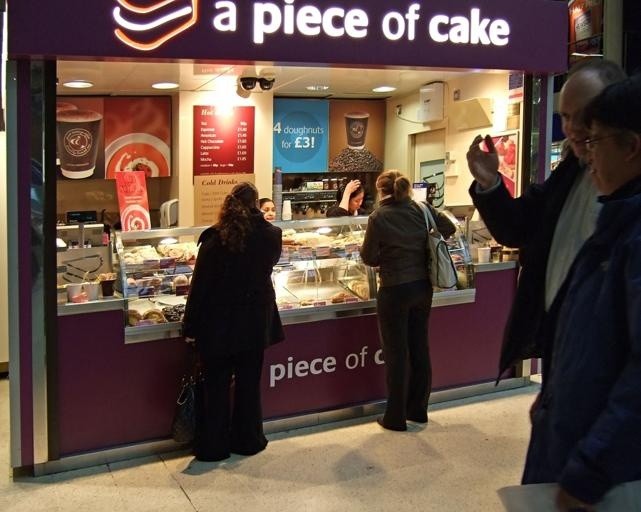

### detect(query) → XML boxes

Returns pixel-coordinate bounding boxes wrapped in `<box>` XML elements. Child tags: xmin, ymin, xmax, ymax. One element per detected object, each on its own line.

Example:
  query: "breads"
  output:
<box><xmin>123</xmin><ymin>228</ymin><xmax>380</xmax><ymax>327</ymax></box>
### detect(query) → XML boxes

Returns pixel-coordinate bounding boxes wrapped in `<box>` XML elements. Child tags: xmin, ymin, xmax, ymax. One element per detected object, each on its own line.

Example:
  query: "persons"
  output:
<box><xmin>468</xmin><ymin>60</ymin><xmax>629</xmax><ymax>490</ymax></box>
<box><xmin>327</xmin><ymin>170</ymin><xmax>456</xmax><ymax>431</ymax></box>
<box><xmin>183</xmin><ymin>182</ymin><xmax>282</xmax><ymax>462</ymax></box>
<box><xmin>517</xmin><ymin>75</ymin><xmax>640</xmax><ymax>512</ymax></box>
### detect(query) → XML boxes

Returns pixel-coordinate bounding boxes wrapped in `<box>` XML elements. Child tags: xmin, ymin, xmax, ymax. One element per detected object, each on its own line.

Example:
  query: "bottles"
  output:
<box><xmin>502</xmin><ymin>249</ymin><xmax>519</xmax><ymax>262</ymax></box>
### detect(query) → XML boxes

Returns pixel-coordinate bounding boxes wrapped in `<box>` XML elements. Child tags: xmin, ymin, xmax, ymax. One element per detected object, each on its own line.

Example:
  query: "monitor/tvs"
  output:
<box><xmin>56</xmin><ymin>224</ymin><xmax>104</xmax><ymax>246</ymax></box>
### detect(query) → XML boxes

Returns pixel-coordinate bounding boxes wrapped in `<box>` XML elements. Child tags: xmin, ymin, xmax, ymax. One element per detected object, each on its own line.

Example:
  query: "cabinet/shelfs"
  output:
<box><xmin>113</xmin><ymin>223</ymin><xmax>465</xmax><ymax>332</ymax></box>
<box><xmin>282</xmin><ymin>188</ymin><xmax>340</xmax><ymax>224</ymax></box>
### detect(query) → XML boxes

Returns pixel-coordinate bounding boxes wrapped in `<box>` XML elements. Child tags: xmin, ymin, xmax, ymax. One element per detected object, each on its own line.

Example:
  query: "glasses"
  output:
<box><xmin>583</xmin><ymin>134</ymin><xmax>614</xmax><ymax>150</ymax></box>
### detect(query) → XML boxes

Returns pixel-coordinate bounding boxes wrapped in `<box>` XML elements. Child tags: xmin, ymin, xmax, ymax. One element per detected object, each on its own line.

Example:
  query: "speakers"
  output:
<box><xmin>448</xmin><ymin>97</ymin><xmax>493</xmax><ymax>131</ymax></box>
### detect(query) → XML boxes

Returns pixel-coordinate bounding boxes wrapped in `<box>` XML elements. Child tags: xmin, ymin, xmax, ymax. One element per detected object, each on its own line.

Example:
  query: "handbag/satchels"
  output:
<box><xmin>415</xmin><ymin>202</ymin><xmax>459</xmax><ymax>288</ymax></box>
<box><xmin>173</xmin><ymin>343</ymin><xmax>204</xmax><ymax>441</ymax></box>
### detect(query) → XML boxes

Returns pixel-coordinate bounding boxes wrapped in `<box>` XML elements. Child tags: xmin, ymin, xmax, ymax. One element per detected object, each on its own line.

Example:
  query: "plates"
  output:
<box><xmin>106</xmin><ymin>132</ymin><xmax>171</xmax><ymax>177</ymax></box>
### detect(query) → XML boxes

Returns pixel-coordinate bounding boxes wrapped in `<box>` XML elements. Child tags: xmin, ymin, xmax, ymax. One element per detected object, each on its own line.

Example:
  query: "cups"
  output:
<box><xmin>84</xmin><ymin>282</ymin><xmax>101</xmax><ymax>300</ymax></box>
<box><xmin>67</xmin><ymin>282</ymin><xmax>83</xmax><ymax>303</ymax></box>
<box><xmin>344</xmin><ymin>111</ymin><xmax>370</xmax><ymax>150</ymax></box>
<box><xmin>54</xmin><ymin>102</ymin><xmax>103</xmax><ymax>180</ymax></box>
<box><xmin>477</xmin><ymin>246</ymin><xmax>501</xmax><ymax>262</ymax></box>
<box><xmin>281</xmin><ymin>199</ymin><xmax>292</xmax><ymax>220</ymax></box>
<box><xmin>100</xmin><ymin>279</ymin><xmax>117</xmax><ymax>298</ymax></box>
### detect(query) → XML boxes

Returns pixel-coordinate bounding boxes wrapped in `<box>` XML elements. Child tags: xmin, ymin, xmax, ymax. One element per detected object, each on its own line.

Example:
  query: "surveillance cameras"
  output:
<box><xmin>240</xmin><ymin>77</ymin><xmax>257</xmax><ymax>90</ymax></box>
<box><xmin>258</xmin><ymin>77</ymin><xmax>275</xmax><ymax>90</ymax></box>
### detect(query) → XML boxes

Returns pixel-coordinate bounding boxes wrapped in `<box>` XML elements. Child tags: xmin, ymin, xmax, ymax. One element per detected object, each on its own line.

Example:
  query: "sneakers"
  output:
<box><xmin>376</xmin><ymin>416</ymin><xmax>406</xmax><ymax>431</ymax></box>
